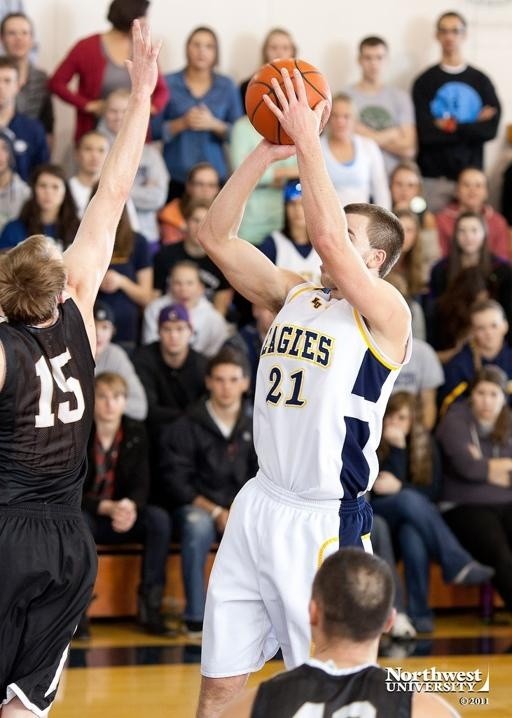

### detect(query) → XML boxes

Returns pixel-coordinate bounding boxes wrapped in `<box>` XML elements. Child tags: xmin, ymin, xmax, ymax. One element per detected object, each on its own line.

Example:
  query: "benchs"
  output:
<box><xmin>84</xmin><ymin>537</ymin><xmax>504</xmax><ymax>619</ymax></box>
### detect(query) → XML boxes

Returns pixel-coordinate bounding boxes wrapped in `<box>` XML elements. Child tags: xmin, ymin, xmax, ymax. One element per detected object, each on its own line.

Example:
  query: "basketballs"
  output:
<box><xmin>245</xmin><ymin>58</ymin><xmax>332</xmax><ymax>145</ymax></box>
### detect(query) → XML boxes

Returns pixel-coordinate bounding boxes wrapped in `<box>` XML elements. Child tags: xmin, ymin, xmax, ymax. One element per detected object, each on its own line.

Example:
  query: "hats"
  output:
<box><xmin>159</xmin><ymin>306</ymin><xmax>188</xmax><ymax>323</ymax></box>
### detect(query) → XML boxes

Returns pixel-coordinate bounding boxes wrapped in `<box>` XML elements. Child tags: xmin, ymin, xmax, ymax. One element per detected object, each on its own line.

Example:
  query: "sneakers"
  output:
<box><xmin>139</xmin><ymin>620</ymin><xmax>202</xmax><ymax>638</ymax></box>
<box><xmin>455</xmin><ymin>563</ymin><xmax>494</xmax><ymax>585</ymax></box>
<box><xmin>74</xmin><ymin>620</ymin><xmax>89</xmax><ymax>640</ymax></box>
<box><xmin>391</xmin><ymin>613</ymin><xmax>432</xmax><ymax>639</ymax></box>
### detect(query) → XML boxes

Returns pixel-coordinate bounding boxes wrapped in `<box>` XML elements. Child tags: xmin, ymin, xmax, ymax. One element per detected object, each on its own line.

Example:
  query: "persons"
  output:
<box><xmin>0</xmin><ymin>0</ymin><xmax>511</xmax><ymax>717</ymax></box>
